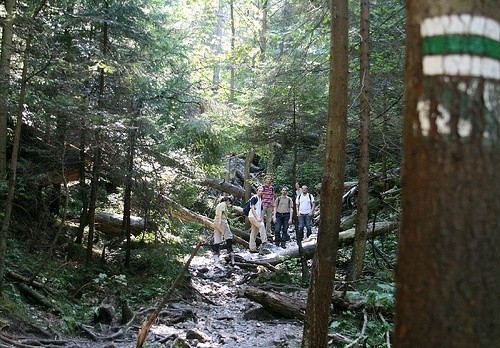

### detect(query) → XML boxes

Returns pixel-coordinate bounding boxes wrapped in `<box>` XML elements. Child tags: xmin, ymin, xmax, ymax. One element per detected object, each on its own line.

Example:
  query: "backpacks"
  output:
<box><xmin>243</xmin><ymin>196</ymin><xmax>257</xmax><ymax>216</ymax></box>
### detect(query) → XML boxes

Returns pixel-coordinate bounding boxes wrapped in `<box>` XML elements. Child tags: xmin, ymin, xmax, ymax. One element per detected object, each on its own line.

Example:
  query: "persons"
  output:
<box><xmin>213</xmin><ymin>197</ymin><xmax>233</xmax><ymax>256</ymax></box>
<box><xmin>272</xmin><ymin>186</ymin><xmax>293</xmax><ymax>249</ymax></box>
<box><xmin>247</xmin><ymin>185</ymin><xmax>271</xmax><ymax>253</ymax></box>
<box><xmin>295</xmin><ymin>183</ymin><xmax>315</xmax><ymax>241</ymax></box>
<box><xmin>261</xmin><ymin>176</ymin><xmax>274</xmax><ymax>237</ymax></box>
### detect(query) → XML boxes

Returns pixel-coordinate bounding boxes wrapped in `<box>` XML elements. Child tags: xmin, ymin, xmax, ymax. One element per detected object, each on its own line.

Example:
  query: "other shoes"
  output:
<box><xmin>281</xmin><ymin>245</ymin><xmax>286</xmax><ymax>249</ymax></box>
<box><xmin>250</xmin><ymin>249</ymin><xmax>258</xmax><ymax>253</ymax></box>
<box><xmin>228</xmin><ymin>251</ymin><xmax>237</xmax><ymax>254</ymax></box>
<box><xmin>263</xmin><ymin>243</ymin><xmax>267</xmax><ymax>247</ymax></box>
